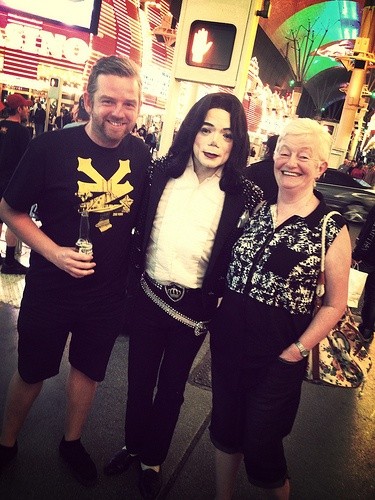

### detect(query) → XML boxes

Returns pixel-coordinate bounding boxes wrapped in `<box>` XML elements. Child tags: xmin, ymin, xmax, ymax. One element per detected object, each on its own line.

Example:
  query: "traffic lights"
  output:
<box><xmin>170</xmin><ymin>0</ymin><xmax>251</xmax><ymax>89</ymax></box>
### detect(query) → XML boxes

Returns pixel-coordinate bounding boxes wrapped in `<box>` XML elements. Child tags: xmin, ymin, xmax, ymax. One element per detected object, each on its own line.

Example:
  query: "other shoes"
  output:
<box><xmin>111</xmin><ymin>445</ymin><xmax>137</xmax><ymax>476</ymax></box>
<box><xmin>0</xmin><ymin>252</ymin><xmax>6</xmax><ymax>266</ymax></box>
<box><xmin>59</xmin><ymin>436</ymin><xmax>97</xmax><ymax>489</ymax></box>
<box><xmin>139</xmin><ymin>461</ymin><xmax>162</xmax><ymax>500</ymax></box>
<box><xmin>0</xmin><ymin>440</ymin><xmax>19</xmax><ymax>495</ymax></box>
<box><xmin>1</xmin><ymin>261</ymin><xmax>30</xmax><ymax>275</ymax></box>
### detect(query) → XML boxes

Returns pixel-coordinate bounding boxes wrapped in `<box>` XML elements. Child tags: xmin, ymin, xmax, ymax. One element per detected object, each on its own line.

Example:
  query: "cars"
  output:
<box><xmin>315</xmin><ymin>168</ymin><xmax>375</xmax><ymax>226</ymax></box>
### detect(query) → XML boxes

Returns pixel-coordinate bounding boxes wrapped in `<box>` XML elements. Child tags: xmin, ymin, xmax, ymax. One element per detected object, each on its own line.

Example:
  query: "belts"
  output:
<box><xmin>145</xmin><ymin>273</ymin><xmax>202</xmax><ymax>301</ymax></box>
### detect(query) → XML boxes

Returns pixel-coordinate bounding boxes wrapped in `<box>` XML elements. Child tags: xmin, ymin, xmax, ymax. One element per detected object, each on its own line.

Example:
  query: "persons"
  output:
<box><xmin>247</xmin><ymin>135</ymin><xmax>280</xmax><ymax>206</ymax></box>
<box><xmin>105</xmin><ymin>90</ymin><xmax>250</xmax><ymax>500</ymax></box>
<box><xmin>33</xmin><ymin>101</ymin><xmax>158</xmax><ymax>156</ymax></box>
<box><xmin>207</xmin><ymin>115</ymin><xmax>352</xmax><ymax>500</ymax></box>
<box><xmin>352</xmin><ymin>204</ymin><xmax>375</xmax><ymax>357</ymax></box>
<box><xmin>0</xmin><ymin>54</ymin><xmax>154</xmax><ymax>483</ymax></box>
<box><xmin>0</xmin><ymin>90</ymin><xmax>35</xmax><ymax>275</ymax></box>
<box><xmin>337</xmin><ymin>158</ymin><xmax>375</xmax><ymax>184</ymax></box>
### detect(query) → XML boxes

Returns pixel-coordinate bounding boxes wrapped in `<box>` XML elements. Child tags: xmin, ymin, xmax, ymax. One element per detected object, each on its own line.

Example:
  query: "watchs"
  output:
<box><xmin>295</xmin><ymin>342</ymin><xmax>309</xmax><ymax>357</ymax></box>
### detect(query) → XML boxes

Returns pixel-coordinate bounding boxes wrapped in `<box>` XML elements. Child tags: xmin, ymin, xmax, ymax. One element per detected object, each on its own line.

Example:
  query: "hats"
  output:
<box><xmin>5</xmin><ymin>94</ymin><xmax>33</xmax><ymax>110</ymax></box>
